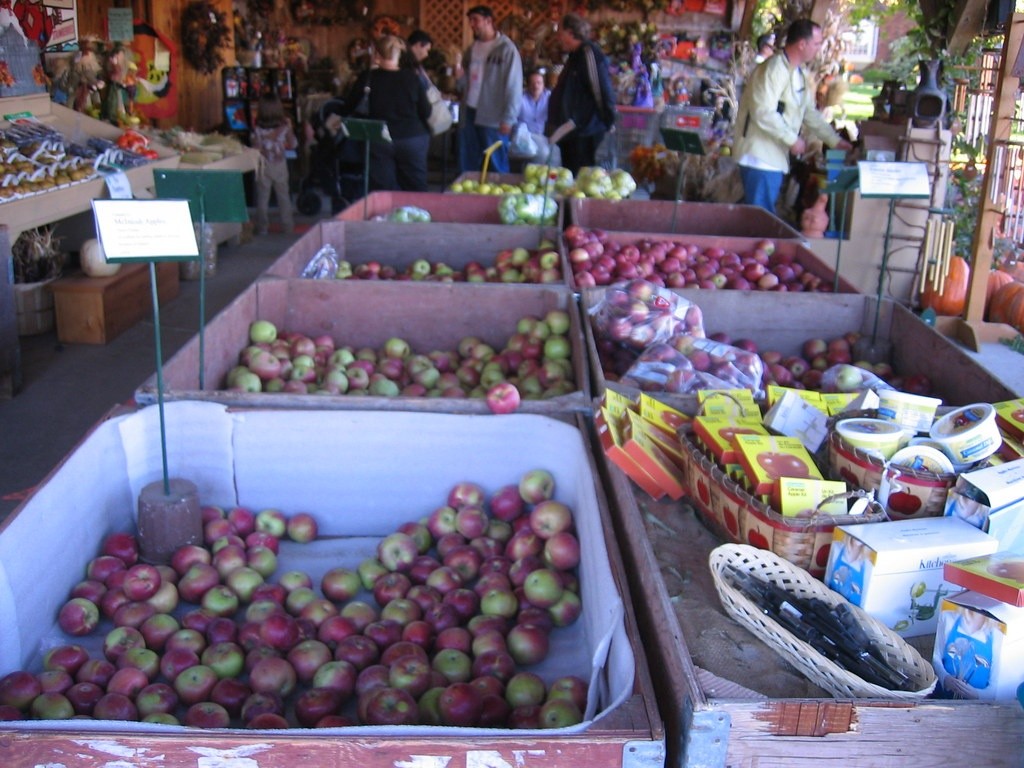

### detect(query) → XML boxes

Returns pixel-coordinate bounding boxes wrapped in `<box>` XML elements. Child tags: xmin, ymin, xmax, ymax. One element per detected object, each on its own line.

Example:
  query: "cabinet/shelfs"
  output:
<box><xmin>793</xmin><ymin>116</ymin><xmax>954</xmax><ymax>303</ymax></box>
<box><xmin>1</xmin><ymin>92</ymin><xmax>266</xmax><ymax>399</ymax></box>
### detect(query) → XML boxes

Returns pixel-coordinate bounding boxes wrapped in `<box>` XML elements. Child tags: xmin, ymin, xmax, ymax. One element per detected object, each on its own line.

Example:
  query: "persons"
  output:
<box><xmin>731</xmin><ymin>19</ymin><xmax>854</xmax><ymax>214</ymax></box>
<box><xmin>72</xmin><ymin>47</ymin><xmax>101</xmax><ymax>113</ymax></box>
<box><xmin>451</xmin><ymin>3</ymin><xmax>519</xmax><ymax>175</ymax></box>
<box><xmin>107</xmin><ymin>44</ymin><xmax>129</xmax><ymax>122</ymax></box>
<box><xmin>314</xmin><ymin>29</ymin><xmax>447</xmax><ymax>190</ymax></box>
<box><xmin>543</xmin><ymin>14</ymin><xmax>618</xmax><ymax>182</ymax></box>
<box><xmin>249</xmin><ymin>92</ymin><xmax>296</xmax><ymax>235</ymax></box>
<box><xmin>748</xmin><ymin>33</ymin><xmax>776</xmax><ymax>76</ymax></box>
<box><xmin>517</xmin><ymin>71</ymin><xmax>553</xmax><ymax>135</ymax></box>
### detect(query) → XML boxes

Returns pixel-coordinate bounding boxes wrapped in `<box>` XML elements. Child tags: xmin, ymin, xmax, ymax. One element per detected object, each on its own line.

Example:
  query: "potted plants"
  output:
<box><xmin>11</xmin><ymin>218</ymin><xmax>70</xmax><ymax>336</ymax></box>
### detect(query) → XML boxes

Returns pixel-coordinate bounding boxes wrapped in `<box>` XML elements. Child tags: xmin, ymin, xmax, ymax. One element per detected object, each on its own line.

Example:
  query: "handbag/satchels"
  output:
<box><xmin>425</xmin><ymin>84</ymin><xmax>453</xmax><ymax>137</ymax></box>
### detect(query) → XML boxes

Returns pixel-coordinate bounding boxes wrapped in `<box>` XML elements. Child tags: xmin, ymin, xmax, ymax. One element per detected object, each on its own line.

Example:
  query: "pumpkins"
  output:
<box><xmin>80</xmin><ymin>236</ymin><xmax>121</xmax><ymax>278</ymax></box>
<box><xmin>918</xmin><ymin>254</ymin><xmax>1024</xmax><ymax>337</ymax></box>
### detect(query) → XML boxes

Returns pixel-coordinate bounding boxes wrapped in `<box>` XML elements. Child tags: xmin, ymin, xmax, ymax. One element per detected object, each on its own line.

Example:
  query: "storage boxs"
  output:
<box><xmin>47</xmin><ymin>257</ymin><xmax>176</xmax><ymax>343</ymax></box>
<box><xmin>559</xmin><ymin>228</ymin><xmax>857</xmax><ymax>288</ymax></box>
<box><xmin>577</xmin><ymin>289</ymin><xmax>1024</xmax><ymax>420</ymax></box>
<box><xmin>133</xmin><ymin>275</ymin><xmax>592</xmax><ymax>416</ymax></box>
<box><xmin>597</xmin><ymin>406</ymin><xmax>1020</xmax><ymax>768</ymax></box>
<box><xmin>571</xmin><ymin>199</ymin><xmax>810</xmax><ymax>248</ymax></box>
<box><xmin>331</xmin><ymin>189</ymin><xmax>564</xmax><ymax>229</ymax></box>
<box><xmin>0</xmin><ymin>396</ymin><xmax>669</xmax><ymax>767</ymax></box>
<box><xmin>257</xmin><ymin>217</ymin><xmax>573</xmax><ymax>290</ymax></box>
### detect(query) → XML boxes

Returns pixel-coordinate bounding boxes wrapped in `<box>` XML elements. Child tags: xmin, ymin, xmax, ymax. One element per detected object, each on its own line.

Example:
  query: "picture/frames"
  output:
<box><xmin>838</xmin><ymin>14</ymin><xmax>880</xmax><ymax>65</ymax></box>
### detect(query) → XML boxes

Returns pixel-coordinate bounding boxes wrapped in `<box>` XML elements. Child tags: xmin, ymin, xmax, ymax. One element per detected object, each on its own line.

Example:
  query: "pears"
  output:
<box><xmin>0</xmin><ymin>136</ymin><xmax>94</xmax><ymax>196</ymax></box>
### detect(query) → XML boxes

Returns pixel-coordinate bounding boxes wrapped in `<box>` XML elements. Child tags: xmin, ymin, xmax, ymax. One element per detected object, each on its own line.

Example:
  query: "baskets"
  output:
<box><xmin>709</xmin><ymin>541</ymin><xmax>938</xmax><ymax>700</ymax></box>
<box><xmin>680</xmin><ymin>391</ymin><xmax>994</xmax><ymax>580</ymax></box>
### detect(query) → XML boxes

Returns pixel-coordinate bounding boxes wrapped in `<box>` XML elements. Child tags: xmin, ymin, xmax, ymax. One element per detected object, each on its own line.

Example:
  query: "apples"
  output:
<box><xmin>587</xmin><ymin>277</ymin><xmax>952</xmax><ymax>407</ymax></box>
<box><xmin>649</xmin><ymin>409</ymin><xmax>922</xmax><ymax>580</ymax></box>
<box><xmin>0</xmin><ymin>468</ymin><xmax>587</xmax><ymax>728</ymax></box>
<box><xmin>368</xmin><ymin>164</ymin><xmax>639</xmax><ymax>227</ymax></box>
<box><xmin>564</xmin><ymin>225</ymin><xmax>836</xmax><ymax>294</ymax></box>
<box><xmin>118</xmin><ymin>132</ymin><xmax>158</xmax><ymax>159</ymax></box>
<box><xmin>224</xmin><ymin>239</ymin><xmax>577</xmax><ymax>414</ymax></box>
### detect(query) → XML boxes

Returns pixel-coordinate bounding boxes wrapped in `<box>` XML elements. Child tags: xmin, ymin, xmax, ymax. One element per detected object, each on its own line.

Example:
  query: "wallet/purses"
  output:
<box><xmin>742</xmin><ymin>99</ymin><xmax>786</xmax><ymax>138</ymax></box>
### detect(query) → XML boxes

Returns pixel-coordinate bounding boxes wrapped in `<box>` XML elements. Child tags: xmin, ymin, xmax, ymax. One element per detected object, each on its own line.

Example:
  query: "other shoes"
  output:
<box><xmin>280</xmin><ymin>223</ymin><xmax>294</xmax><ymax>233</ymax></box>
<box><xmin>254</xmin><ymin>228</ymin><xmax>269</xmax><ymax>236</ymax></box>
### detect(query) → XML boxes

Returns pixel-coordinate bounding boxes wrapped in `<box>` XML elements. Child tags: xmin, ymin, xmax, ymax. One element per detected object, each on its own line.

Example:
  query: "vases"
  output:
<box><xmin>867</xmin><ymin>58</ymin><xmax>948</xmax><ymax>127</ymax></box>
